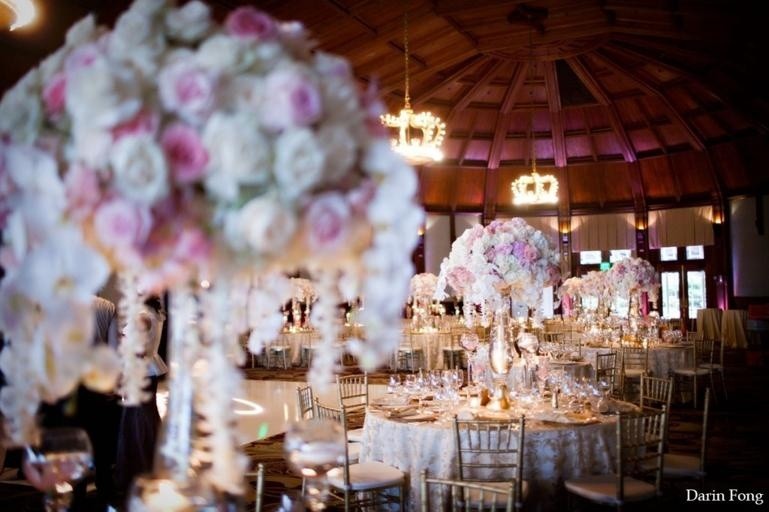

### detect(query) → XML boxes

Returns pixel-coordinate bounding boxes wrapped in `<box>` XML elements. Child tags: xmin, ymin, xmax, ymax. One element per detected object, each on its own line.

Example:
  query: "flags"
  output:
<box><xmin>628</xmin><ymin>294</ymin><xmax>640</xmax><ymax>340</ymax></box>
<box><xmin>156</xmin><ymin>280</ymin><xmax>220</xmax><ymax>512</ymax></box>
<box><xmin>490</xmin><ymin>310</ymin><xmax>513</xmax><ymax>407</ymax></box>
<box><xmin>595</xmin><ymin>300</ymin><xmax>606</xmax><ymax>319</ymax></box>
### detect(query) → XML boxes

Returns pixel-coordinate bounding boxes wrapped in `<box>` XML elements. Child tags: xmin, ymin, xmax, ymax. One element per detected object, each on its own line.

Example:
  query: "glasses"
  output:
<box><xmin>638</xmin><ymin>230</ymin><xmax>645</xmax><ymax>245</ymax></box>
<box><xmin>381</xmin><ymin>0</ymin><xmax>446</xmax><ymax>162</ymax></box>
<box><xmin>562</xmin><ymin>233</ymin><xmax>569</xmax><ymax>248</ymax></box>
<box><xmin>510</xmin><ymin>12</ymin><xmax>558</xmax><ymax>205</ymax></box>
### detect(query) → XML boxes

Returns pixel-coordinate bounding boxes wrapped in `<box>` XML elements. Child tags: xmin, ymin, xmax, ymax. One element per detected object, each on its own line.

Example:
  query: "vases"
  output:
<box><xmin>156</xmin><ymin>280</ymin><xmax>220</xmax><ymax>512</ymax></box>
<box><xmin>613</xmin><ymin>258</ymin><xmax>661</xmax><ymax>307</ymax></box>
<box><xmin>434</xmin><ymin>216</ymin><xmax>560</xmax><ymax>327</ymax></box>
<box><xmin>0</xmin><ymin>0</ymin><xmax>424</xmax><ymax>493</ymax></box>
<box><xmin>490</xmin><ymin>310</ymin><xmax>513</xmax><ymax>407</ymax></box>
<box><xmin>628</xmin><ymin>294</ymin><xmax>640</xmax><ymax>340</ymax></box>
<box><xmin>595</xmin><ymin>300</ymin><xmax>606</xmax><ymax>319</ymax></box>
<box><xmin>578</xmin><ymin>271</ymin><xmax>611</xmax><ymax>297</ymax></box>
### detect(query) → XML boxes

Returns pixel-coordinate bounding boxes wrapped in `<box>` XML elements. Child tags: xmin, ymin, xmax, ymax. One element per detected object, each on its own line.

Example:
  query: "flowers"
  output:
<box><xmin>578</xmin><ymin>271</ymin><xmax>611</xmax><ymax>297</ymax></box>
<box><xmin>434</xmin><ymin>216</ymin><xmax>560</xmax><ymax>327</ymax></box>
<box><xmin>0</xmin><ymin>0</ymin><xmax>424</xmax><ymax>493</ymax></box>
<box><xmin>613</xmin><ymin>258</ymin><xmax>661</xmax><ymax>307</ymax></box>
<box><xmin>246</xmin><ymin>326</ymin><xmax>725</xmax><ymax>512</ymax></box>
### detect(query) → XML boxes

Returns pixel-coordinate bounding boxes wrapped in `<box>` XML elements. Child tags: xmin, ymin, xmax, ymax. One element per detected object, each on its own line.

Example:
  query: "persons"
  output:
<box><xmin>113</xmin><ymin>285</ymin><xmax>166</xmax><ymax>498</ymax></box>
<box><xmin>72</xmin><ymin>296</ymin><xmax>121</xmax><ymax>487</ymax></box>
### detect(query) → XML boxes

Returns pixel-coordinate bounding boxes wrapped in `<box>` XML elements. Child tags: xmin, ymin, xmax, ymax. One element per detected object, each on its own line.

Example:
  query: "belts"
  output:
<box><xmin>22</xmin><ymin>430</ymin><xmax>93</xmax><ymax>511</ymax></box>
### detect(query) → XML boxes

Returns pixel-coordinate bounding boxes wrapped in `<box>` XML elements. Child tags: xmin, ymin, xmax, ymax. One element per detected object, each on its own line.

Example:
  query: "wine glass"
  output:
<box><xmin>280</xmin><ymin>309</ymin><xmax>685</xmax><ymax>428</ymax></box>
<box><xmin>22</xmin><ymin>430</ymin><xmax>93</xmax><ymax>511</ymax></box>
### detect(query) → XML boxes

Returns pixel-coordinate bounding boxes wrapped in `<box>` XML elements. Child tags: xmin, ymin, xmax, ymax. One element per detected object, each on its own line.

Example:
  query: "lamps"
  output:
<box><xmin>510</xmin><ymin>12</ymin><xmax>558</xmax><ymax>205</ymax></box>
<box><xmin>638</xmin><ymin>230</ymin><xmax>645</xmax><ymax>245</ymax></box>
<box><xmin>381</xmin><ymin>0</ymin><xmax>446</xmax><ymax>162</ymax></box>
<box><xmin>562</xmin><ymin>233</ymin><xmax>569</xmax><ymax>248</ymax></box>
